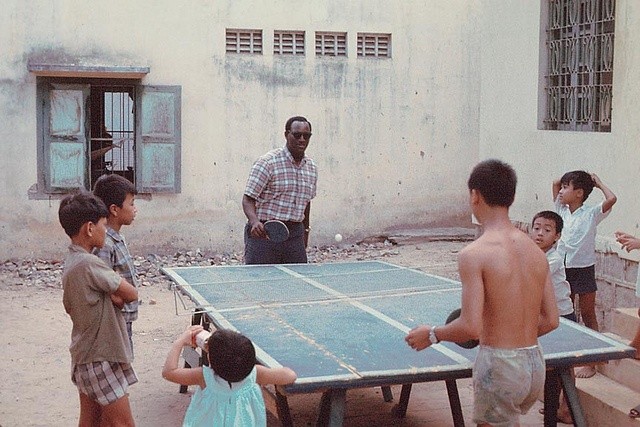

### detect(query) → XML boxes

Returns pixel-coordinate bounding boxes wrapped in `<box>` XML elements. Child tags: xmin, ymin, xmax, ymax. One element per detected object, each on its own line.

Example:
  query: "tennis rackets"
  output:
<box><xmin>445</xmin><ymin>308</ymin><xmax>479</xmax><ymax>348</ymax></box>
<box><xmin>263</xmin><ymin>219</ymin><xmax>290</xmax><ymax>243</ymax></box>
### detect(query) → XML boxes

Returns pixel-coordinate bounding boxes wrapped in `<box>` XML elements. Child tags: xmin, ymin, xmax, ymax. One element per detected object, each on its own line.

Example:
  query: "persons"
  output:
<box><xmin>241</xmin><ymin>116</ymin><xmax>319</xmax><ymax>264</ymax></box>
<box><xmin>550</xmin><ymin>169</ymin><xmax>617</xmax><ymax>377</ymax></box>
<box><xmin>404</xmin><ymin>158</ymin><xmax>560</xmax><ymax>425</ymax></box>
<box><xmin>92</xmin><ymin>174</ymin><xmax>140</xmax><ymax>363</ymax></box>
<box><xmin>57</xmin><ymin>191</ymin><xmax>138</xmax><ymax>427</ymax></box>
<box><xmin>161</xmin><ymin>325</ymin><xmax>297</xmax><ymax>427</ymax></box>
<box><xmin>530</xmin><ymin>209</ymin><xmax>576</xmax><ymax>425</ymax></box>
<box><xmin>614</xmin><ymin>230</ymin><xmax>640</xmax><ymax>417</ymax></box>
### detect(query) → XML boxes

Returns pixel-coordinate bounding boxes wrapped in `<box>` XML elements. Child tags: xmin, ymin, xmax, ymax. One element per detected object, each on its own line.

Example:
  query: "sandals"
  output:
<box><xmin>629</xmin><ymin>405</ymin><xmax>640</xmax><ymax>418</ymax></box>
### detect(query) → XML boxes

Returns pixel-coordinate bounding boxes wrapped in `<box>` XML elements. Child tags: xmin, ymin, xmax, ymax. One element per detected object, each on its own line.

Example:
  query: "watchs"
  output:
<box><xmin>428</xmin><ymin>326</ymin><xmax>440</xmax><ymax>344</ymax></box>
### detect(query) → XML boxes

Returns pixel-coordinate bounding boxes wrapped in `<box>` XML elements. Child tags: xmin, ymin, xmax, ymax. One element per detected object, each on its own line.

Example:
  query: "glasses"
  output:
<box><xmin>291</xmin><ymin>132</ymin><xmax>312</xmax><ymax>139</ymax></box>
